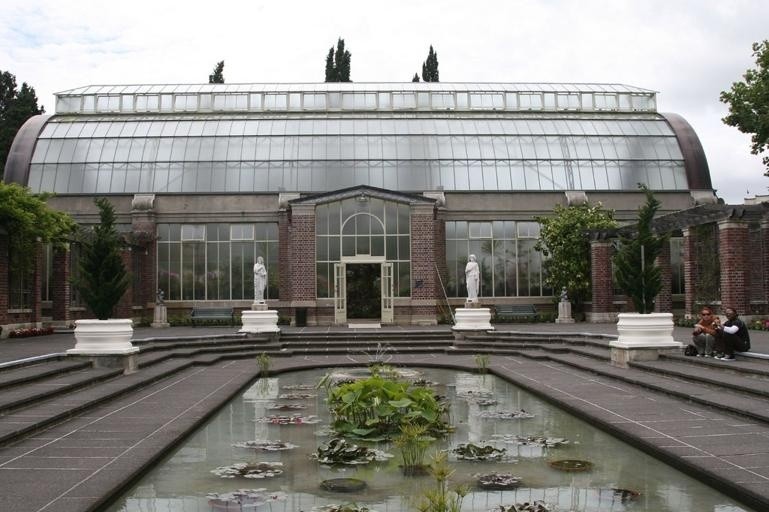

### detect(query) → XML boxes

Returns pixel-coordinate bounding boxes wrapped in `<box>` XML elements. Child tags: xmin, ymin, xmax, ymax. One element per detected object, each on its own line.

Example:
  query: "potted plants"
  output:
<box><xmin>67</xmin><ymin>194</ymin><xmax>134</xmax><ymax>351</ymax></box>
<box><xmin>610</xmin><ymin>181</ymin><xmax>675</xmax><ymax>344</ymax></box>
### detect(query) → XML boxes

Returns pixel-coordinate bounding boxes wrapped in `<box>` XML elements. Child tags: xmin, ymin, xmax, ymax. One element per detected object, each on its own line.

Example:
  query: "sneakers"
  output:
<box><xmin>696</xmin><ymin>352</ymin><xmax>736</xmax><ymax>361</ymax></box>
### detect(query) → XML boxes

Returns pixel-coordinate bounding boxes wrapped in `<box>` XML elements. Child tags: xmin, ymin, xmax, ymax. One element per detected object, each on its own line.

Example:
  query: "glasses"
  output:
<box><xmin>702</xmin><ymin>312</ymin><xmax>712</xmax><ymax>317</ymax></box>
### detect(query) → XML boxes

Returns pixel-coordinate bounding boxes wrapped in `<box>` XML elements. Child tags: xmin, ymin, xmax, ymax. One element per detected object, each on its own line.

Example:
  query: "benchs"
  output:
<box><xmin>190</xmin><ymin>305</ymin><xmax>234</xmax><ymax>329</ymax></box>
<box><xmin>494</xmin><ymin>303</ymin><xmax>541</xmax><ymax>323</ymax></box>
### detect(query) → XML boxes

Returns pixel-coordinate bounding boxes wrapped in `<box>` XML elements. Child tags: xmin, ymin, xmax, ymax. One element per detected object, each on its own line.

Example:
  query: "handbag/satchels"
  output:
<box><xmin>684</xmin><ymin>345</ymin><xmax>698</xmax><ymax>357</ymax></box>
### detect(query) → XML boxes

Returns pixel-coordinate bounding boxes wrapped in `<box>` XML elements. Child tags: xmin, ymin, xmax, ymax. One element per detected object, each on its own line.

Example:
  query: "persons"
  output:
<box><xmin>155</xmin><ymin>288</ymin><xmax>165</xmax><ymax>304</ymax></box>
<box><xmin>712</xmin><ymin>308</ymin><xmax>751</xmax><ymax>361</ymax></box>
<box><xmin>465</xmin><ymin>254</ymin><xmax>480</xmax><ymax>303</ymax></box>
<box><xmin>559</xmin><ymin>285</ymin><xmax>569</xmax><ymax>302</ymax></box>
<box><xmin>253</xmin><ymin>256</ymin><xmax>267</xmax><ymax>304</ymax></box>
<box><xmin>692</xmin><ymin>308</ymin><xmax>721</xmax><ymax>357</ymax></box>
